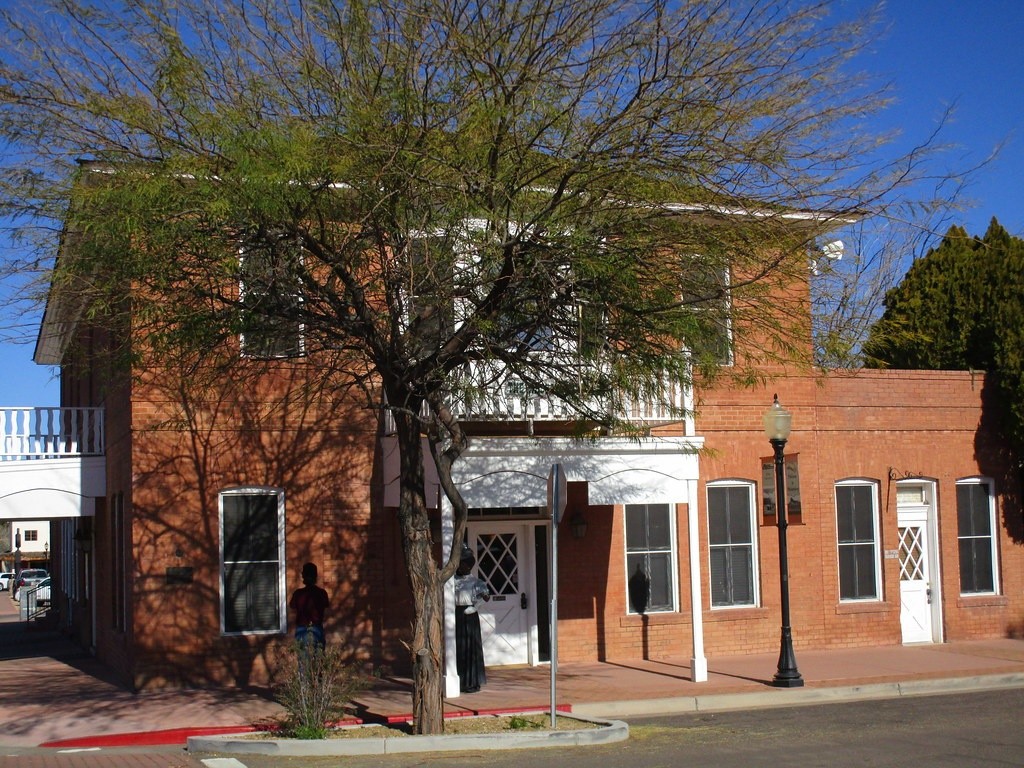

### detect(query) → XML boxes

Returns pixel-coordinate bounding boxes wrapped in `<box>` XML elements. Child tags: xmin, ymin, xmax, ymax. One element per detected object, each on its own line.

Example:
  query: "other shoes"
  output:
<box><xmin>460</xmin><ymin>685</ymin><xmax>481</xmax><ymax>693</ymax></box>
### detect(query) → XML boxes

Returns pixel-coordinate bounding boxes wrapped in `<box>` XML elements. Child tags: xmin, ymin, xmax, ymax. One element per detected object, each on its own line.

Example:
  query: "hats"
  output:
<box><xmin>460</xmin><ymin>543</ymin><xmax>473</xmax><ymax>560</ymax></box>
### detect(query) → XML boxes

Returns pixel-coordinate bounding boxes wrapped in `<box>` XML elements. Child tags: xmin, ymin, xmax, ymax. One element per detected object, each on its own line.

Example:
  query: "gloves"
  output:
<box><xmin>464</xmin><ymin>597</ymin><xmax>487</xmax><ymax>614</ymax></box>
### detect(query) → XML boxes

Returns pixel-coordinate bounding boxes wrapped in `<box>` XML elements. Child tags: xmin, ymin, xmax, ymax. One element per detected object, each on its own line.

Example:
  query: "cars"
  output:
<box><xmin>13</xmin><ymin>569</ymin><xmax>51</xmax><ymax>602</ymax></box>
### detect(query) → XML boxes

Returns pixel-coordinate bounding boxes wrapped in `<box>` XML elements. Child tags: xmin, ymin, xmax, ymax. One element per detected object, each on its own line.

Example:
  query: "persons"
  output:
<box><xmin>290</xmin><ymin>562</ymin><xmax>330</xmax><ymax>676</ymax></box>
<box><xmin>456</xmin><ymin>546</ymin><xmax>490</xmax><ymax>694</ymax></box>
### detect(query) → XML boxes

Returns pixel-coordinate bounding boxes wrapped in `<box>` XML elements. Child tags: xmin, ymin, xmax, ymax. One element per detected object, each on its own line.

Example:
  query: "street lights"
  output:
<box><xmin>763</xmin><ymin>395</ymin><xmax>806</xmax><ymax>688</ymax></box>
<box><xmin>44</xmin><ymin>541</ymin><xmax>50</xmax><ymax>571</ymax></box>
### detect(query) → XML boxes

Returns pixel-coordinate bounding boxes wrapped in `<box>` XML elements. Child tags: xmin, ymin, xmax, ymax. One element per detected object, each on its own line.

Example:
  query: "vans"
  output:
<box><xmin>0</xmin><ymin>573</ymin><xmax>15</xmax><ymax>592</ymax></box>
